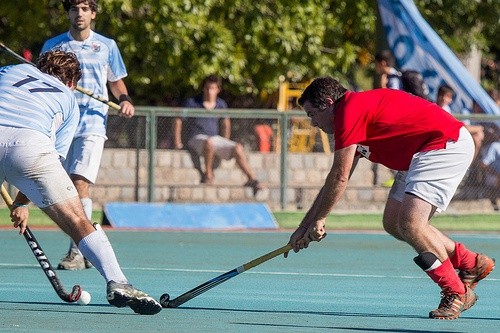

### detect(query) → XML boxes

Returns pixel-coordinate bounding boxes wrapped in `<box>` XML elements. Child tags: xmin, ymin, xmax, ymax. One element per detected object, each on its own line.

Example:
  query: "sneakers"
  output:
<box><xmin>430</xmin><ymin>284</ymin><xmax>479</xmax><ymax>319</ymax></box>
<box><xmin>105</xmin><ymin>279</ymin><xmax>162</xmax><ymax>314</ymax></box>
<box><xmin>459</xmin><ymin>252</ymin><xmax>494</xmax><ymax>289</ymax></box>
<box><xmin>57</xmin><ymin>248</ymin><xmax>93</xmax><ymax>270</ymax></box>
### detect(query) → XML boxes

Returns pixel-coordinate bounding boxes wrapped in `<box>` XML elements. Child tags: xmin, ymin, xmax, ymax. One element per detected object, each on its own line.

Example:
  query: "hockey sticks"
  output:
<box><xmin>160</xmin><ymin>237</ymin><xmax>305</xmax><ymax>309</ymax></box>
<box><xmin>0</xmin><ymin>42</ymin><xmax>122</xmax><ymax>112</ymax></box>
<box><xmin>1</xmin><ymin>184</ymin><xmax>82</xmax><ymax>302</ymax></box>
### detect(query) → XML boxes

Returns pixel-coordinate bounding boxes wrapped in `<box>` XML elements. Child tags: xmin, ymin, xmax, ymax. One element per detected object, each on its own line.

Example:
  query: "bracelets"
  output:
<box><xmin>118</xmin><ymin>94</ymin><xmax>133</xmax><ymax>105</ymax></box>
<box><xmin>12</xmin><ymin>202</ymin><xmax>29</xmax><ymax>207</ymax></box>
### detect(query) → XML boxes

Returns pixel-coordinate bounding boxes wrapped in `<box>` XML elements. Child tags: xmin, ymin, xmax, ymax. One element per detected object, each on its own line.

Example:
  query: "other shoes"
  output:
<box><xmin>202</xmin><ymin>172</ymin><xmax>213</xmax><ymax>183</ymax></box>
<box><xmin>245</xmin><ymin>179</ymin><xmax>262</xmax><ymax>191</ymax></box>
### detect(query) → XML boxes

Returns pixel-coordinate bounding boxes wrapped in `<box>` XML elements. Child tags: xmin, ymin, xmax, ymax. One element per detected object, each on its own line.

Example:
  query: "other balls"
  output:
<box><xmin>77</xmin><ymin>290</ymin><xmax>91</xmax><ymax>305</ymax></box>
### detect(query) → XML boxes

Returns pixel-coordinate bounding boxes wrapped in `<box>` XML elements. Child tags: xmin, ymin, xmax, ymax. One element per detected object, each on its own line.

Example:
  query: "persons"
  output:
<box><xmin>38</xmin><ymin>0</ymin><xmax>135</xmax><ymax>271</ymax></box>
<box><xmin>0</xmin><ymin>47</ymin><xmax>164</xmax><ymax>315</ymax></box>
<box><xmin>436</xmin><ymin>85</ymin><xmax>485</xmax><ymax>186</ymax></box>
<box><xmin>175</xmin><ymin>79</ymin><xmax>263</xmax><ymax>189</ymax></box>
<box><xmin>283</xmin><ymin>78</ymin><xmax>495</xmax><ymax>321</ymax></box>
<box><xmin>375</xmin><ymin>52</ymin><xmax>402</xmax><ymax>90</ymax></box>
